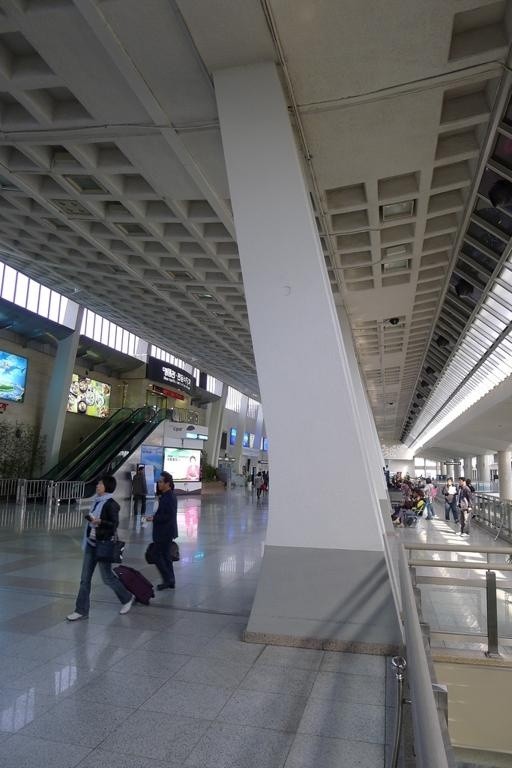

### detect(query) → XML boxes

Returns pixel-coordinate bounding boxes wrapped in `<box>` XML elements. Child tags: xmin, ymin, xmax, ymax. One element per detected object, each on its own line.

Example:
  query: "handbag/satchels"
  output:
<box><xmin>145</xmin><ymin>542</ymin><xmax>180</xmax><ymax>564</ymax></box>
<box><xmin>95</xmin><ymin>540</ymin><xmax>126</xmax><ymax>563</ymax></box>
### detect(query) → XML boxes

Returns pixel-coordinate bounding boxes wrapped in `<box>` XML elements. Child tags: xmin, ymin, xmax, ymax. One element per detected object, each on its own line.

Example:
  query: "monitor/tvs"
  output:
<box><xmin>163</xmin><ymin>446</ymin><xmax>202</xmax><ymax>482</ymax></box>
<box><xmin>0</xmin><ymin>349</ymin><xmax>30</xmax><ymax>404</ymax></box>
<box><xmin>230</xmin><ymin>427</ymin><xmax>268</xmax><ymax>452</ymax></box>
<box><xmin>67</xmin><ymin>372</ymin><xmax>113</xmax><ymax>419</ymax></box>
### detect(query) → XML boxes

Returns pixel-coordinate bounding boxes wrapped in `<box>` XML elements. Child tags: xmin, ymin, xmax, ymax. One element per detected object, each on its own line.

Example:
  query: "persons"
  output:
<box><xmin>67</xmin><ymin>476</ymin><xmax>136</xmax><ymax>621</ymax></box>
<box><xmin>383</xmin><ymin>464</ymin><xmax>438</xmax><ymax>528</ymax></box>
<box><xmin>132</xmin><ymin>467</ymin><xmax>148</xmax><ymax>516</ymax></box>
<box><xmin>442</xmin><ymin>477</ymin><xmax>475</xmax><ymax>537</ymax></box>
<box><xmin>255</xmin><ymin>471</ymin><xmax>269</xmax><ymax>499</ymax></box>
<box><xmin>146</xmin><ymin>471</ymin><xmax>178</xmax><ymax>590</ymax></box>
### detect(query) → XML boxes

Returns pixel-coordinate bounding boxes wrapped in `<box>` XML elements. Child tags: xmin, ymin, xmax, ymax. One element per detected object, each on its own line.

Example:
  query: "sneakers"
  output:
<box><xmin>67</xmin><ymin>610</ymin><xmax>89</xmax><ymax>621</ymax></box>
<box><xmin>120</xmin><ymin>593</ymin><xmax>136</xmax><ymax>614</ymax></box>
<box><xmin>456</xmin><ymin>532</ymin><xmax>470</xmax><ymax>537</ymax></box>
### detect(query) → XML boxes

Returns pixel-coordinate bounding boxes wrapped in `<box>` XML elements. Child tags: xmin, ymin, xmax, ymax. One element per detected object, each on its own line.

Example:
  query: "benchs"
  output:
<box><xmin>403</xmin><ymin>502</ymin><xmax>429</xmax><ymax>527</ymax></box>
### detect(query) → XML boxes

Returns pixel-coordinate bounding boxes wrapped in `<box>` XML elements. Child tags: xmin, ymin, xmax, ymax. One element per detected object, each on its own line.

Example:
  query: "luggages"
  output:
<box><xmin>112</xmin><ymin>566</ymin><xmax>155</xmax><ymax>605</ymax></box>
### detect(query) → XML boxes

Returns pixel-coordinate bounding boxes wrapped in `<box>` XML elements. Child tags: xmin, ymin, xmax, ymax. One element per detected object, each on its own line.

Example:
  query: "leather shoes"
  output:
<box><xmin>157</xmin><ymin>582</ymin><xmax>175</xmax><ymax>591</ymax></box>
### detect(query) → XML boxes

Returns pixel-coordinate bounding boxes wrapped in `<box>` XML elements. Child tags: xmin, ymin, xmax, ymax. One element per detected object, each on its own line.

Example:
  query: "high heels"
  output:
<box><xmin>392</xmin><ymin>520</ymin><xmax>404</xmax><ymax>528</ymax></box>
